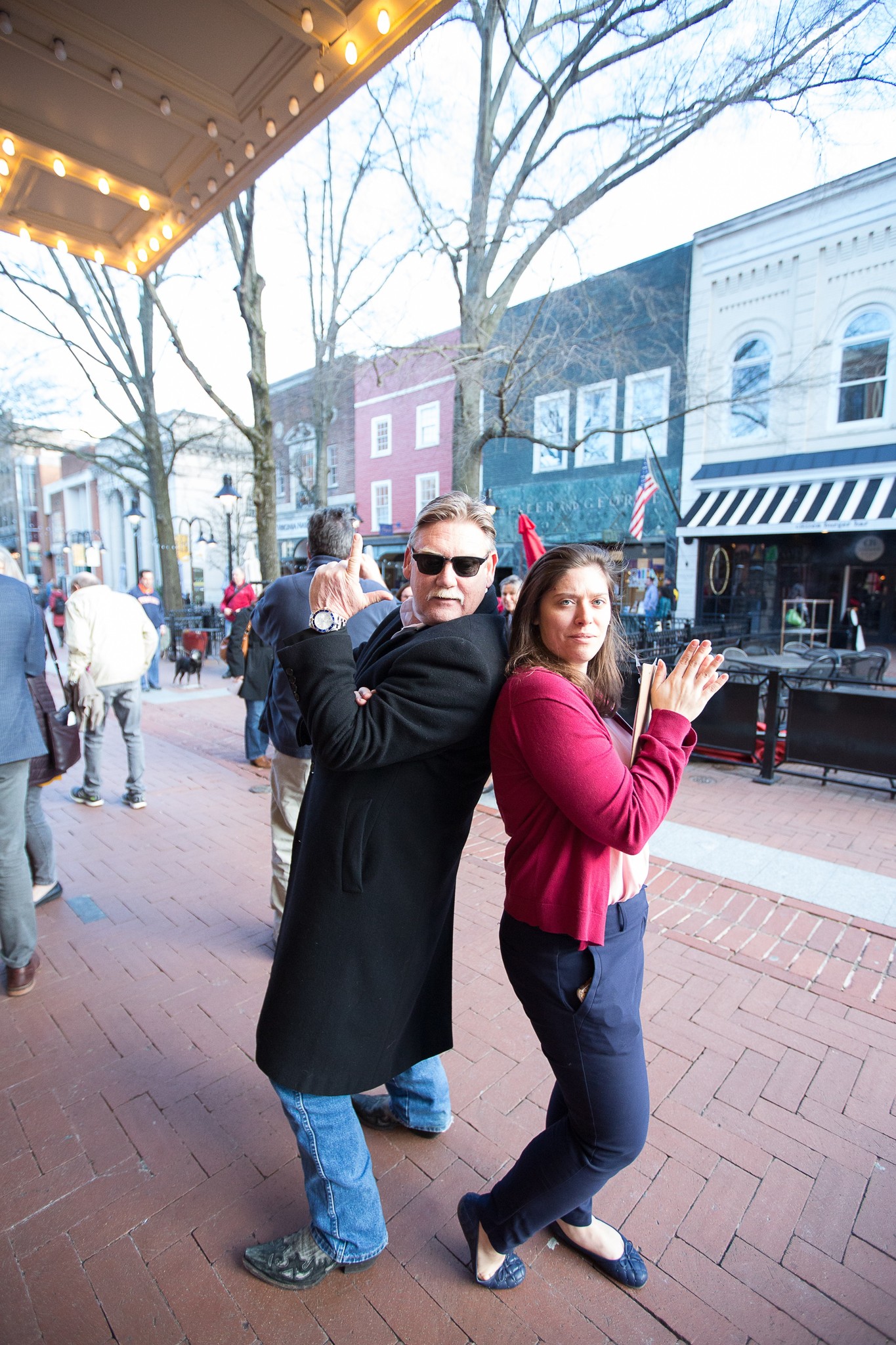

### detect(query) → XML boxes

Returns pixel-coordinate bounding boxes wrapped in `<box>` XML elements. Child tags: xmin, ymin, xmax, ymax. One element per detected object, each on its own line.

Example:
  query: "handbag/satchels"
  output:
<box><xmin>46</xmin><ymin>701</ymin><xmax>81</xmax><ymax>773</ymax></box>
<box><xmin>219</xmin><ymin>631</ymin><xmax>248</xmax><ymax>663</ymax></box>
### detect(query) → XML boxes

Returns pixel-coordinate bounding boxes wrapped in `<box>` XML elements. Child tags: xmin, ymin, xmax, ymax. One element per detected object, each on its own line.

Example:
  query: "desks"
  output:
<box><xmin>727</xmin><ymin>653</ymin><xmax>837</xmax><ymax>684</ymax></box>
<box><xmin>808</xmin><ymin>647</ymin><xmax>859</xmax><ymax>659</ymax></box>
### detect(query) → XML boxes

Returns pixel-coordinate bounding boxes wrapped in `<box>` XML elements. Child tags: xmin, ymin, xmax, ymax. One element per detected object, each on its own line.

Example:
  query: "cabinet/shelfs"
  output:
<box><xmin>780</xmin><ymin>598</ymin><xmax>834</xmax><ymax>655</ymax></box>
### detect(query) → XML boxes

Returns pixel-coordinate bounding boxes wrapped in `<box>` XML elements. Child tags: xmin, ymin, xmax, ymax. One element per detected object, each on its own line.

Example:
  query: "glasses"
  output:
<box><xmin>410</xmin><ymin>547</ymin><xmax>494</xmax><ymax>577</ymax></box>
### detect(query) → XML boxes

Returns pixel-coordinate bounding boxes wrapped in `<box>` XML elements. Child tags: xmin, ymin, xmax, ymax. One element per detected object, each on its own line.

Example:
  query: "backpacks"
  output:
<box><xmin>55</xmin><ymin>598</ymin><xmax>65</xmax><ymax>614</ymax></box>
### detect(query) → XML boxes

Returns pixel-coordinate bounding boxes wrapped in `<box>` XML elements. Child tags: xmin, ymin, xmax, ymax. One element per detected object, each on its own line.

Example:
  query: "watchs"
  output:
<box><xmin>308</xmin><ymin>607</ymin><xmax>347</xmax><ymax>633</ymax></box>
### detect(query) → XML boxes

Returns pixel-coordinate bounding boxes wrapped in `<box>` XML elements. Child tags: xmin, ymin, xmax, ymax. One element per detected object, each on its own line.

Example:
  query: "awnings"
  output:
<box><xmin>0</xmin><ymin>0</ymin><xmax>463</xmax><ymax>281</ymax></box>
<box><xmin>675</xmin><ymin>444</ymin><xmax>896</xmax><ymax>546</ymax></box>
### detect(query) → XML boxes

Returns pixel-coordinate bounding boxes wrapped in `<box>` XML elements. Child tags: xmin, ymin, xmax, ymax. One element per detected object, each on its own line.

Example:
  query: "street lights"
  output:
<box><xmin>121</xmin><ymin>497</ymin><xmax>148</xmax><ymax>586</ymax></box>
<box><xmin>213</xmin><ymin>473</ymin><xmax>243</xmax><ymax>637</ymax></box>
<box><xmin>154</xmin><ymin>514</ymin><xmax>219</xmax><ymax>603</ymax></box>
<box><xmin>61</xmin><ymin>530</ymin><xmax>108</xmax><ymax>576</ymax></box>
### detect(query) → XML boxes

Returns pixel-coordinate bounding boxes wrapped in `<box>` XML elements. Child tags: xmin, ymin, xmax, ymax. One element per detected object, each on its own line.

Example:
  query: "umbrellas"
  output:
<box><xmin>518</xmin><ymin>509</ymin><xmax>547</xmax><ymax>570</ymax></box>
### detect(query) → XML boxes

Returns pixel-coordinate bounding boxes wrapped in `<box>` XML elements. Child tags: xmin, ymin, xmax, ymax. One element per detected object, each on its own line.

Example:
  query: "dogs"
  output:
<box><xmin>168</xmin><ymin>649</ymin><xmax>202</xmax><ymax>688</ymax></box>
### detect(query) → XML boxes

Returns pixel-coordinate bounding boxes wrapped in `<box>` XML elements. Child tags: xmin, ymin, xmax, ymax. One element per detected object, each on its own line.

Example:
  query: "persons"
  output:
<box><xmin>0</xmin><ymin>544</ymin><xmax>63</xmax><ymax>996</ymax></box>
<box><xmin>241</xmin><ymin>491</ymin><xmax>510</xmax><ymax>1291</ymax></box>
<box><xmin>736</xmin><ymin>580</ymin><xmax>874</xmax><ymax>628</ymax></box>
<box><xmin>31</xmin><ymin>578</ymin><xmax>68</xmax><ymax>647</ymax></box>
<box><xmin>226</xmin><ymin>582</ymin><xmax>275</xmax><ymax>768</ymax></box>
<box><xmin>395</xmin><ymin>581</ymin><xmax>413</xmax><ymax>602</ymax></box>
<box><xmin>499</xmin><ymin>574</ymin><xmax>524</xmax><ymax>634</ymax></box>
<box><xmin>186</xmin><ymin>594</ymin><xmax>191</xmax><ymax>604</ymax></box>
<box><xmin>127</xmin><ymin>569</ymin><xmax>167</xmax><ymax>692</ymax></box>
<box><xmin>456</xmin><ymin>544</ymin><xmax>729</xmax><ymax>1290</ymax></box>
<box><xmin>220</xmin><ymin>568</ymin><xmax>257</xmax><ymax>679</ymax></box>
<box><xmin>250</xmin><ymin>507</ymin><xmax>402</xmax><ymax>948</ymax></box>
<box><xmin>643</xmin><ymin>577</ymin><xmax>679</xmax><ymax>633</ymax></box>
<box><xmin>63</xmin><ymin>571</ymin><xmax>159</xmax><ymax>809</ymax></box>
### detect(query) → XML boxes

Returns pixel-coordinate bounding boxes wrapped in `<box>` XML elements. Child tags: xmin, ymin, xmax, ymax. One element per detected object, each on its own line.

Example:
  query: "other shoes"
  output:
<box><xmin>455</xmin><ymin>1191</ymin><xmax>525</xmax><ymax>1288</ymax></box>
<box><xmin>351</xmin><ymin>1092</ymin><xmax>444</xmax><ymax>1140</ymax></box>
<box><xmin>68</xmin><ymin>786</ymin><xmax>103</xmax><ymax>807</ymax></box>
<box><xmin>121</xmin><ymin>791</ymin><xmax>148</xmax><ymax>810</ymax></box>
<box><xmin>551</xmin><ymin>1213</ymin><xmax>649</xmax><ymax>1289</ymax></box>
<box><xmin>249</xmin><ymin>754</ymin><xmax>273</xmax><ymax>769</ymax></box>
<box><xmin>242</xmin><ymin>1227</ymin><xmax>379</xmax><ymax>1290</ymax></box>
<box><xmin>32</xmin><ymin>880</ymin><xmax>63</xmax><ymax>909</ymax></box>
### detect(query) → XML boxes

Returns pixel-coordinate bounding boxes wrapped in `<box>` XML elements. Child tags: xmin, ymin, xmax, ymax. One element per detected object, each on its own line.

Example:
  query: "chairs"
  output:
<box><xmin>670</xmin><ymin>642</ymin><xmax>892</xmax><ymax>724</ymax></box>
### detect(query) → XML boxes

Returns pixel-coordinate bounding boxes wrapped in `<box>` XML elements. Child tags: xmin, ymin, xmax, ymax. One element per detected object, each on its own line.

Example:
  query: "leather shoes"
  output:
<box><xmin>5</xmin><ymin>949</ymin><xmax>41</xmax><ymax>996</ymax></box>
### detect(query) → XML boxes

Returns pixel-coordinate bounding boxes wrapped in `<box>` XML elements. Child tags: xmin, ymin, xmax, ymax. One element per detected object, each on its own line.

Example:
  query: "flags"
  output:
<box><xmin>629</xmin><ymin>453</ymin><xmax>660</xmax><ymax>541</ymax></box>
